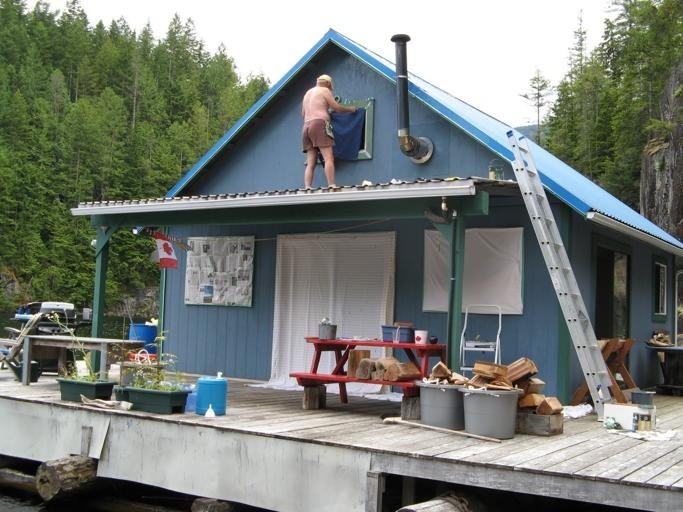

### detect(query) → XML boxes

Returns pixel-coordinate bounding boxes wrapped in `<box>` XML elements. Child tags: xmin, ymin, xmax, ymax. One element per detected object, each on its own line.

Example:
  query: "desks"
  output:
<box><xmin>20</xmin><ymin>332</ymin><xmax>146</xmax><ymax>387</ymax></box>
<box><xmin>304</xmin><ymin>336</ymin><xmax>447</xmax><ymax>404</ymax></box>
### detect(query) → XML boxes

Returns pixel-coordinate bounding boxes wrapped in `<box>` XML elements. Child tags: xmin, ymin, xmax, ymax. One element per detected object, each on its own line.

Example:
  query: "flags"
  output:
<box><xmin>151</xmin><ymin>230</ymin><xmax>179</xmax><ymax>269</ymax></box>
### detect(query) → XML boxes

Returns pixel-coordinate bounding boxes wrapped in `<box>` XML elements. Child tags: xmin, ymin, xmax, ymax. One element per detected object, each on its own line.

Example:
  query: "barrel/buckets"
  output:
<box><xmin>128</xmin><ymin>322</ymin><xmax>158</xmax><ymax>354</ymax></box>
<box><xmin>630</xmin><ymin>390</ymin><xmax>658</xmax><ymax>408</ymax></box>
<box><xmin>318</xmin><ymin>317</ymin><xmax>337</xmax><ymax>340</ymax></box>
<box><xmin>197</xmin><ymin>371</ymin><xmax>227</xmax><ymax>416</ymax></box>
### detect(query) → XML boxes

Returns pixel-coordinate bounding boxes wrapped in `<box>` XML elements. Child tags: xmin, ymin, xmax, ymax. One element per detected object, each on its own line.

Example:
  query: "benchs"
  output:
<box><xmin>288</xmin><ymin>371</ymin><xmax>421</xmax><ymax>421</ymax></box>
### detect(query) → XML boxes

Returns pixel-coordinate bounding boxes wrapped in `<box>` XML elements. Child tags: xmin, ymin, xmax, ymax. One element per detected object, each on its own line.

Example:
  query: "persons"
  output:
<box><xmin>300</xmin><ymin>74</ymin><xmax>358</xmax><ymax>190</ymax></box>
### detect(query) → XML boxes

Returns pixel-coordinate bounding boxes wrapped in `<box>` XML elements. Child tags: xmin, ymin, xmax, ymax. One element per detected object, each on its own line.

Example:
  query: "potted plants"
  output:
<box><xmin>44</xmin><ymin>309</ymin><xmax>192</xmax><ymax>415</ymax></box>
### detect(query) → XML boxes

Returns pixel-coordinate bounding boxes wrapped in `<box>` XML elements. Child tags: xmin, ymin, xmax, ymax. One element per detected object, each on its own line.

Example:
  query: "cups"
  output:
<box><xmin>392</xmin><ymin>329</ymin><xmax>400</xmax><ymax>343</ymax></box>
<box><xmin>414</xmin><ymin>330</ymin><xmax>428</xmax><ymax>344</ymax></box>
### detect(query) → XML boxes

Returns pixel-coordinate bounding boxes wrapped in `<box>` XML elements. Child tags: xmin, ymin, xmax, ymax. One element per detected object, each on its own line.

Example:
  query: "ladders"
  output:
<box><xmin>506</xmin><ymin>129</ymin><xmax>613</xmax><ymax>422</ymax></box>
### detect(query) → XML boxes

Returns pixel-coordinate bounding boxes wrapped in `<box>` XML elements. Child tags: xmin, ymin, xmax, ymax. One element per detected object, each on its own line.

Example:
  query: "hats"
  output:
<box><xmin>317</xmin><ymin>74</ymin><xmax>333</xmax><ymax>90</ymax></box>
